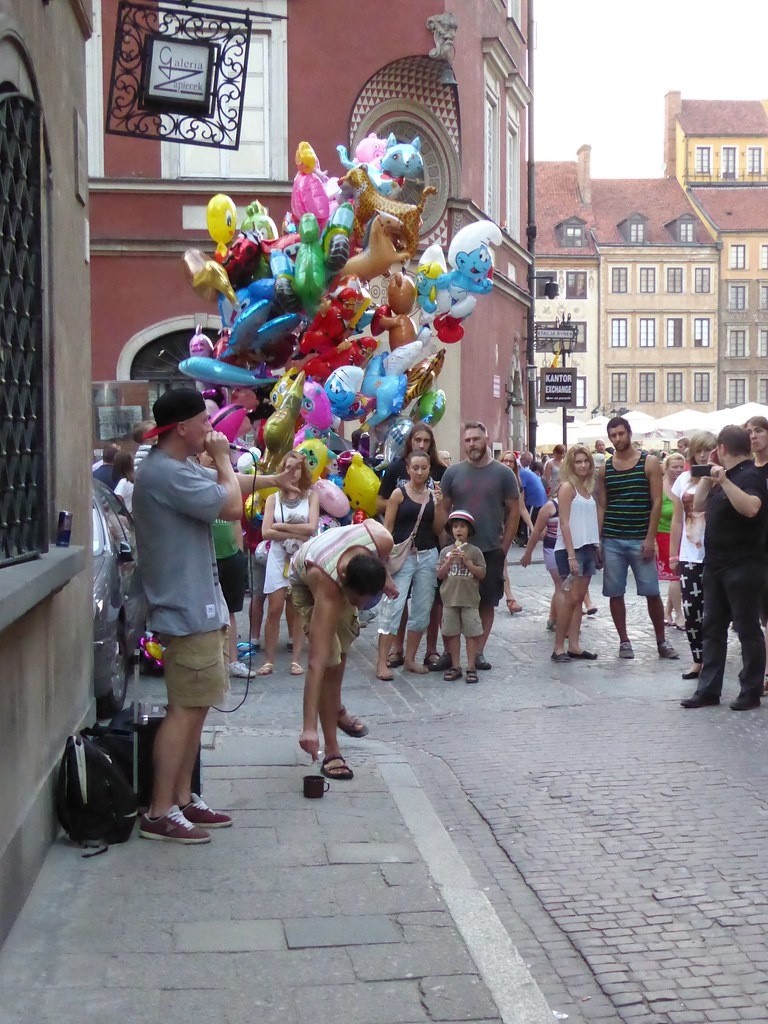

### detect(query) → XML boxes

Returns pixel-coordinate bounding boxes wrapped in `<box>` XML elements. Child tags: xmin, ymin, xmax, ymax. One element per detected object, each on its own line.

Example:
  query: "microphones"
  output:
<box><xmin>229</xmin><ymin>443</ymin><xmax>250</xmax><ymax>452</ymax></box>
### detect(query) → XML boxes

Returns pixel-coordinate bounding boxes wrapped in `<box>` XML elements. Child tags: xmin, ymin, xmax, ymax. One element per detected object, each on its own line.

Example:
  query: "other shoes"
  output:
<box><xmin>429</xmin><ymin>650</ymin><xmax>452</xmax><ymax>671</ymax></box>
<box><xmin>567</xmin><ymin>650</ymin><xmax>597</xmax><ymax>660</ymax></box>
<box><xmin>619</xmin><ymin>639</ymin><xmax>634</xmax><ymax>658</ymax></box>
<box><xmin>763</xmin><ymin>674</ymin><xmax>768</xmax><ymax>695</ymax></box>
<box><xmin>244</xmin><ymin>641</ymin><xmax>260</xmax><ymax>652</ymax></box>
<box><xmin>682</xmin><ymin>670</ymin><xmax>698</xmax><ymax>679</ymax></box>
<box><xmin>286</xmin><ymin>643</ymin><xmax>294</xmax><ymax>652</ymax></box>
<box><xmin>680</xmin><ymin>694</ymin><xmax>720</xmax><ymax>708</ymax></box>
<box><xmin>658</xmin><ymin>641</ymin><xmax>679</xmax><ymax>659</ymax></box>
<box><xmin>551</xmin><ymin>652</ymin><xmax>570</xmax><ymax>662</ymax></box>
<box><xmin>587</xmin><ymin>608</ymin><xmax>597</xmax><ymax>615</ymax></box>
<box><xmin>728</xmin><ymin>693</ymin><xmax>761</xmax><ymax>711</ymax></box>
<box><xmin>476</xmin><ymin>650</ymin><xmax>492</xmax><ymax>670</ymax></box>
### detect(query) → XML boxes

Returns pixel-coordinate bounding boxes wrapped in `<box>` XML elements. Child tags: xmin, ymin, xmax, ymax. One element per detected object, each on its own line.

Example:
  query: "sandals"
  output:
<box><xmin>444</xmin><ymin>668</ymin><xmax>463</xmax><ymax>681</ymax></box>
<box><xmin>321</xmin><ymin>753</ymin><xmax>354</xmax><ymax>780</ymax></box>
<box><xmin>386</xmin><ymin>652</ymin><xmax>404</xmax><ymax>667</ymax></box>
<box><xmin>466</xmin><ymin>670</ymin><xmax>478</xmax><ymax>683</ymax></box>
<box><xmin>258</xmin><ymin>663</ymin><xmax>274</xmax><ymax>674</ymax></box>
<box><xmin>337</xmin><ymin>705</ymin><xmax>368</xmax><ymax>738</ymax></box>
<box><xmin>546</xmin><ymin>620</ymin><xmax>557</xmax><ymax>631</ymax></box>
<box><xmin>291</xmin><ymin>662</ymin><xmax>303</xmax><ymax>675</ymax></box>
<box><xmin>506</xmin><ymin>599</ymin><xmax>522</xmax><ymax>615</ymax></box>
<box><xmin>424</xmin><ymin>651</ymin><xmax>441</xmax><ymax>665</ymax></box>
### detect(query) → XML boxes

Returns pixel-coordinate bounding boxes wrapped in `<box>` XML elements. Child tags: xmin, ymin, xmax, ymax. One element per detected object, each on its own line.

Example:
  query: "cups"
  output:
<box><xmin>304</xmin><ymin>775</ymin><xmax>331</xmax><ymax>798</ymax></box>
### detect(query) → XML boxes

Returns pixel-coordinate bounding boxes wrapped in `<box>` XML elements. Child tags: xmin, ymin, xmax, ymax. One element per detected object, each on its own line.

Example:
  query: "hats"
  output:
<box><xmin>143</xmin><ymin>388</ymin><xmax>206</xmax><ymax>438</ymax></box>
<box><xmin>445</xmin><ymin>510</ymin><xmax>475</xmax><ymax>538</ymax></box>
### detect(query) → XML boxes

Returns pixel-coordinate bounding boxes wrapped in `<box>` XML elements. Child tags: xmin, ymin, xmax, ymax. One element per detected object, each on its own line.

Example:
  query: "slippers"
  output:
<box><xmin>676</xmin><ymin>625</ymin><xmax>686</xmax><ymax>631</ymax></box>
<box><xmin>664</xmin><ymin>619</ymin><xmax>676</xmax><ymax>626</ymax></box>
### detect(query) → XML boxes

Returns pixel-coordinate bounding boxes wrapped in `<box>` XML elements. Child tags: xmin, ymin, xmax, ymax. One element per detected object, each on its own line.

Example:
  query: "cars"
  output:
<box><xmin>92</xmin><ymin>474</ymin><xmax>148</xmax><ymax>716</ymax></box>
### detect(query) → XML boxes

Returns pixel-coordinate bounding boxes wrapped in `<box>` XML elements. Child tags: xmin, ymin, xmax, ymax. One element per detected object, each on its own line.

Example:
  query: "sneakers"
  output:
<box><xmin>140</xmin><ymin>805</ymin><xmax>211</xmax><ymax>843</ymax></box>
<box><xmin>229</xmin><ymin>662</ymin><xmax>256</xmax><ymax>679</ymax></box>
<box><xmin>180</xmin><ymin>793</ymin><xmax>233</xmax><ymax>828</ymax></box>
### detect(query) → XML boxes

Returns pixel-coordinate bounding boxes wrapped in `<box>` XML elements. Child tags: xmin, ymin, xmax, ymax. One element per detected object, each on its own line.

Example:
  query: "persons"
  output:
<box><xmin>90</xmin><ymin>418</ymin><xmax>768</xmax><ymax>847</ymax></box>
<box><xmin>132</xmin><ymin>388</ymin><xmax>307</xmax><ymax>844</ymax></box>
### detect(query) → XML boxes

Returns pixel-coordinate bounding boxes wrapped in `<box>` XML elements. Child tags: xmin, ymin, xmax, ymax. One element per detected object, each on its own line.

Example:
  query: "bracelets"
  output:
<box><xmin>568</xmin><ymin>556</ymin><xmax>575</xmax><ymax>560</ymax></box>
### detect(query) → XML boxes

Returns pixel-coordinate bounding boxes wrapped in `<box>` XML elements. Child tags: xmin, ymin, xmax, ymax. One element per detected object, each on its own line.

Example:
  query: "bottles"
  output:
<box><xmin>377</xmin><ymin>596</ymin><xmax>393</xmax><ymax>635</ymax></box>
<box><xmin>561</xmin><ymin>564</ymin><xmax>579</xmax><ymax>593</ymax></box>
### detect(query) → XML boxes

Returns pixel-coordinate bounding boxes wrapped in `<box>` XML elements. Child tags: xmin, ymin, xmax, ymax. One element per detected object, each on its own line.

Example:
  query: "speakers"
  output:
<box><xmin>138</xmin><ymin>702</ymin><xmax>201</xmax><ymax>809</ymax></box>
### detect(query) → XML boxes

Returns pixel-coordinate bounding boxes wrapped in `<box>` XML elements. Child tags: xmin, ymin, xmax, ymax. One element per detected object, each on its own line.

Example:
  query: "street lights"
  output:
<box><xmin>559</xmin><ymin>312</ymin><xmax>579</xmax><ymax>453</ymax></box>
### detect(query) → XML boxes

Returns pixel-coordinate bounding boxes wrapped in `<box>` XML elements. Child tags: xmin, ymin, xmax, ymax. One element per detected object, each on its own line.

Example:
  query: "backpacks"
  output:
<box><xmin>54</xmin><ymin>734</ymin><xmax>139</xmax><ymax>857</ymax></box>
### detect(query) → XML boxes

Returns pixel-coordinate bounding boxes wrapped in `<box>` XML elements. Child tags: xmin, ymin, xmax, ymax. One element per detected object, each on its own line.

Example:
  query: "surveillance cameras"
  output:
<box><xmin>544</xmin><ymin>282</ymin><xmax>558</xmax><ymax>299</ymax></box>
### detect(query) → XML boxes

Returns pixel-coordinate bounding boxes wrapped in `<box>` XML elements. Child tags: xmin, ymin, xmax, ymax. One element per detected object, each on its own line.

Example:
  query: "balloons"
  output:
<box><xmin>238</xmin><ymin>641</ymin><xmax>258</xmax><ymax>662</ymax></box>
<box><xmin>139</xmin><ymin>631</ymin><xmax>166</xmax><ymax>670</ymax></box>
<box><xmin>179</xmin><ymin>132</ymin><xmax>503</xmax><ymax>567</ymax></box>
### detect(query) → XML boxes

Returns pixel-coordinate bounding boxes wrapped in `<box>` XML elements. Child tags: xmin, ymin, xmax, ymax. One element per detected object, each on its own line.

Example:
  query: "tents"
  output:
<box><xmin>535</xmin><ymin>401</ymin><xmax>768</xmax><ymax>449</ymax></box>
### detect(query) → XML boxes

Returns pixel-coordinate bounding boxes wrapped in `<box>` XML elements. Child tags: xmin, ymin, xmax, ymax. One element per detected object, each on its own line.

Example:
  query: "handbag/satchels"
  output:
<box><xmin>387</xmin><ymin>536</ymin><xmax>414</xmax><ymax>575</ymax></box>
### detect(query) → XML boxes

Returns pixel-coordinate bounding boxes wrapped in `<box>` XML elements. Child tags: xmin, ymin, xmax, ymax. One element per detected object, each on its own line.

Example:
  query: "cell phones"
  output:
<box><xmin>690</xmin><ymin>465</ymin><xmax>712</xmax><ymax>477</ymax></box>
